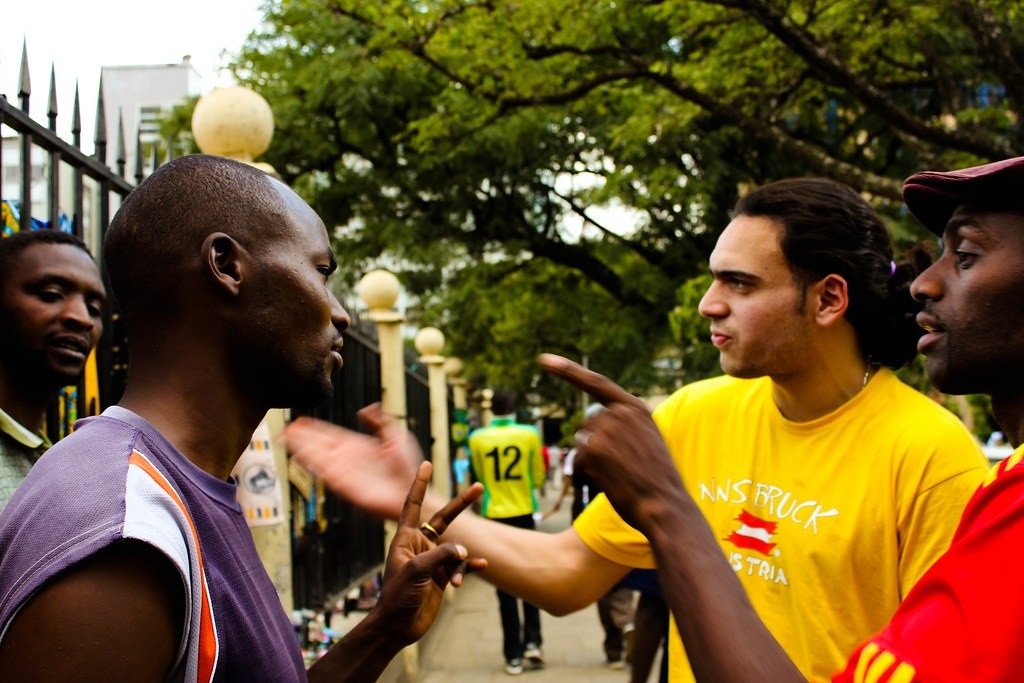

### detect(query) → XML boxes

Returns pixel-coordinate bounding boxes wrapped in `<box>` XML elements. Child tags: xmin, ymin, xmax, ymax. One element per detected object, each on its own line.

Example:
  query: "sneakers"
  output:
<box><xmin>524</xmin><ymin>642</ymin><xmax>543</xmax><ymax>667</ymax></box>
<box><xmin>506</xmin><ymin>658</ymin><xmax>523</xmax><ymax>674</ymax></box>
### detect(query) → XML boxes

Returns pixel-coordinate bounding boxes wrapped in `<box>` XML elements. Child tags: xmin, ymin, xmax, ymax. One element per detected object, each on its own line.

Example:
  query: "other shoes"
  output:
<box><xmin>622</xmin><ymin>623</ymin><xmax>634</xmax><ymax>664</ymax></box>
<box><xmin>610</xmin><ymin>661</ymin><xmax>623</xmax><ymax>668</ymax></box>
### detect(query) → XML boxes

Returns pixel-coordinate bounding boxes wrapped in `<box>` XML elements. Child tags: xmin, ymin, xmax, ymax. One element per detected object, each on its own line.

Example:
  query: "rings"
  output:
<box><xmin>421</xmin><ymin>523</ymin><xmax>439</xmax><ymax>538</ymax></box>
<box><xmin>575</xmin><ymin>429</ymin><xmax>592</xmax><ymax>446</ymax></box>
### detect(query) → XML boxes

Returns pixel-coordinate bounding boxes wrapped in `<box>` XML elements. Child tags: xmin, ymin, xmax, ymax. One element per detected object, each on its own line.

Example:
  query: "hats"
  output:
<box><xmin>902</xmin><ymin>157</ymin><xmax>1023</xmax><ymax>238</ymax></box>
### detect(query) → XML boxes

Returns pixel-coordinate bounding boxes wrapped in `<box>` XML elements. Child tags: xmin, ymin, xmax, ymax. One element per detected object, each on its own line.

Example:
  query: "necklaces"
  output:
<box><xmin>863</xmin><ymin>364</ymin><xmax>870</xmax><ymax>386</ymax></box>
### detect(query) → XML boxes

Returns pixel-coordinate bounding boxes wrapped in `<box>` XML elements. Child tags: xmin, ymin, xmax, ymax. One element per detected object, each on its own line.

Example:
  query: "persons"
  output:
<box><xmin>538</xmin><ymin>404</ymin><xmax>668</xmax><ymax>683</ymax></box>
<box><xmin>0</xmin><ymin>155</ymin><xmax>485</xmax><ymax>683</ymax></box>
<box><xmin>469</xmin><ymin>385</ymin><xmax>544</xmax><ymax>673</ymax></box>
<box><xmin>0</xmin><ymin>230</ymin><xmax>108</xmax><ymax>512</ymax></box>
<box><xmin>537</xmin><ymin>156</ymin><xmax>1024</xmax><ymax>683</ymax></box>
<box><xmin>284</xmin><ymin>176</ymin><xmax>989</xmax><ymax>683</ymax></box>
